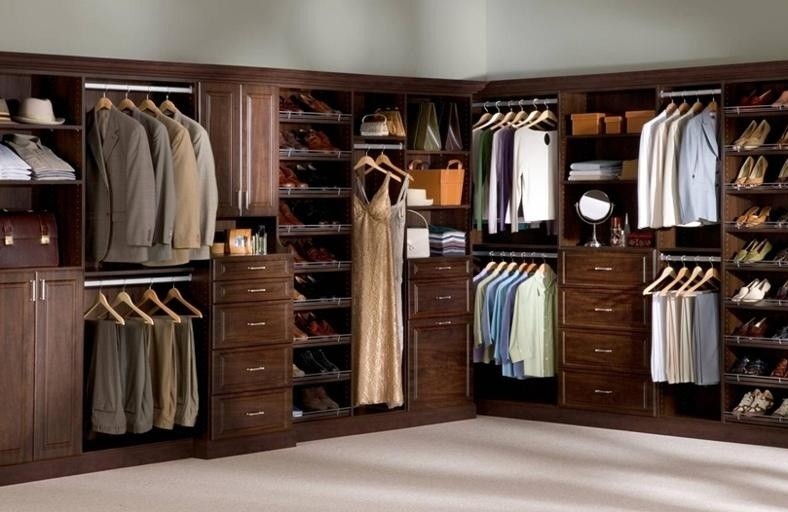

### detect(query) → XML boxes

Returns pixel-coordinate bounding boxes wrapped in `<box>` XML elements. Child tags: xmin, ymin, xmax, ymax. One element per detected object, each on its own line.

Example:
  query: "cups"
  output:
<box><xmin>256</xmin><ymin>232</ymin><xmax>267</xmax><ymax>255</ymax></box>
<box><xmin>244</xmin><ymin>235</ymin><xmax>256</xmax><ymax>255</ymax></box>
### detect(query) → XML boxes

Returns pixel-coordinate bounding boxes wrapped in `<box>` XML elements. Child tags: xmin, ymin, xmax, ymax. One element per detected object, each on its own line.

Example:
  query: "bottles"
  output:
<box><xmin>258</xmin><ymin>224</ymin><xmax>266</xmax><ymax>235</ymax></box>
<box><xmin>611</xmin><ymin>217</ymin><xmax>623</xmax><ymax>249</ymax></box>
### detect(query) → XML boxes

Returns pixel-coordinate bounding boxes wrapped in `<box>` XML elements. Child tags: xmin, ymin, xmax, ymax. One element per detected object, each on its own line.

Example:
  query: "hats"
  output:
<box><xmin>13</xmin><ymin>97</ymin><xmax>67</xmax><ymax>125</ymax></box>
<box><xmin>0</xmin><ymin>97</ymin><xmax>16</xmax><ymax>125</ymax></box>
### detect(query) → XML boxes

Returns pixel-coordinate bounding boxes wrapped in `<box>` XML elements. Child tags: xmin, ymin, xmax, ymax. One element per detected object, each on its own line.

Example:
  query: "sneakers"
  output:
<box><xmin>730</xmin><ymin>389</ymin><xmax>761</xmax><ymax>417</ymax></box>
<box><xmin>770</xmin><ymin>91</ymin><xmax>787</xmax><ymax>107</ymax></box>
<box><xmin>278</xmin><ymin>91</ymin><xmax>342</xmax><ymax>115</ymax></box>
<box><xmin>770</xmin><ymin>398</ymin><xmax>787</xmax><ymax>418</ymax></box>
<box><xmin>314</xmin><ymin>387</ymin><xmax>340</xmax><ymax>411</ymax></box>
<box><xmin>299</xmin><ymin>388</ymin><xmax>327</xmax><ymax>411</ymax></box>
<box><xmin>278</xmin><ymin>202</ymin><xmax>343</xmax><ymax>227</ymax></box>
<box><xmin>279</xmin><ymin>164</ymin><xmax>330</xmax><ymax>188</ymax></box>
<box><xmin>293</xmin><ymin>275</ymin><xmax>323</xmax><ymax>300</ymax></box>
<box><xmin>305</xmin><ymin>274</ymin><xmax>336</xmax><ymax>299</ymax></box>
<box><xmin>278</xmin><ymin>129</ymin><xmax>340</xmax><ymax>155</ymax></box>
<box><xmin>774</xmin><ymin>280</ymin><xmax>787</xmax><ymax>300</ymax></box>
<box><xmin>296</xmin><ymin>313</ymin><xmax>327</xmax><ymax>338</ymax></box>
<box><xmin>769</xmin><ymin>357</ymin><xmax>787</xmax><ymax>378</ymax></box>
<box><xmin>300</xmin><ymin>349</ymin><xmax>328</xmax><ymax>375</ymax></box>
<box><xmin>306</xmin><ymin>239</ymin><xmax>340</xmax><ymax>261</ymax></box>
<box><xmin>771</xmin><ymin>247</ymin><xmax>787</xmax><ymax>263</ymax></box>
<box><xmin>314</xmin><ymin>314</ymin><xmax>339</xmax><ymax>340</ymax></box>
<box><xmin>294</xmin><ymin>321</ymin><xmax>309</xmax><ymax>341</ymax></box>
<box><xmin>293</xmin><ymin>361</ymin><xmax>306</xmax><ymax>378</ymax></box>
<box><xmin>743</xmin><ymin>387</ymin><xmax>775</xmax><ymax>418</ymax></box>
<box><xmin>316</xmin><ymin>347</ymin><xmax>339</xmax><ymax>372</ymax></box>
<box><xmin>770</xmin><ymin>325</ymin><xmax>787</xmax><ymax>339</ymax></box>
<box><xmin>283</xmin><ymin>243</ymin><xmax>305</xmax><ymax>264</ymax></box>
<box><xmin>743</xmin><ymin>356</ymin><xmax>766</xmax><ymax>376</ymax></box>
<box><xmin>295</xmin><ymin>238</ymin><xmax>331</xmax><ymax>264</ymax></box>
<box><xmin>776</xmin><ymin>120</ymin><xmax>787</xmax><ymax>146</ymax></box>
<box><xmin>731</xmin><ymin>356</ymin><xmax>751</xmax><ymax>376</ymax></box>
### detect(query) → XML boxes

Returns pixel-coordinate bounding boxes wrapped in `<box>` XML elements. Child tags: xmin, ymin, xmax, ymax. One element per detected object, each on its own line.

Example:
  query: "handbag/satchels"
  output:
<box><xmin>407</xmin><ymin>210</ymin><xmax>430</xmax><ymax>258</ymax></box>
<box><xmin>359</xmin><ymin>114</ymin><xmax>389</xmax><ymax>136</ymax></box>
<box><xmin>372</xmin><ymin>110</ymin><xmax>405</xmax><ymax>136</ymax></box>
<box><xmin>2</xmin><ymin>206</ymin><xmax>60</xmax><ymax>269</ymax></box>
<box><xmin>408</xmin><ymin>101</ymin><xmax>442</xmax><ymax>151</ymax></box>
<box><xmin>441</xmin><ymin>102</ymin><xmax>463</xmax><ymax>152</ymax></box>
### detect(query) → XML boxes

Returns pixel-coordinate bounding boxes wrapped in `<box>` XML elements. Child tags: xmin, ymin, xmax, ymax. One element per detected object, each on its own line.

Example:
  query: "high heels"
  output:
<box><xmin>733</xmin><ymin>156</ymin><xmax>753</xmax><ymax>187</ymax></box>
<box><xmin>735</xmin><ymin>206</ymin><xmax>759</xmax><ymax>224</ymax></box>
<box><xmin>744</xmin><ymin>155</ymin><xmax>768</xmax><ymax>187</ymax></box>
<box><xmin>776</xmin><ymin>157</ymin><xmax>787</xmax><ymax>186</ymax></box>
<box><xmin>751</xmin><ymin>88</ymin><xmax>772</xmax><ymax>105</ymax></box>
<box><xmin>731</xmin><ymin>121</ymin><xmax>756</xmax><ymax>150</ymax></box>
<box><xmin>744</xmin><ymin>120</ymin><xmax>770</xmax><ymax>150</ymax></box>
<box><xmin>744</xmin><ymin>206</ymin><xmax>772</xmax><ymax>224</ymax></box>
<box><xmin>739</xmin><ymin>90</ymin><xmax>755</xmax><ymax>105</ymax></box>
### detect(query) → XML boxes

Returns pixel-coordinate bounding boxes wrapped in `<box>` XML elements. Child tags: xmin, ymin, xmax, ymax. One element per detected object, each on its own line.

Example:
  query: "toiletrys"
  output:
<box><xmin>610</xmin><ymin>213</ymin><xmax>630</xmax><ymax>247</ymax></box>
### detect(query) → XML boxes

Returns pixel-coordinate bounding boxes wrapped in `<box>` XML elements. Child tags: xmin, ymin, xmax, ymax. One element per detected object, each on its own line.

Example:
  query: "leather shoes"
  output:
<box><xmin>741</xmin><ymin>238</ymin><xmax>773</xmax><ymax>263</ymax></box>
<box><xmin>733</xmin><ymin>238</ymin><xmax>759</xmax><ymax>263</ymax></box>
<box><xmin>741</xmin><ymin>278</ymin><xmax>771</xmax><ymax>302</ymax></box>
<box><xmin>731</xmin><ymin>317</ymin><xmax>756</xmax><ymax>337</ymax></box>
<box><xmin>751</xmin><ymin>317</ymin><xmax>769</xmax><ymax>337</ymax></box>
<box><xmin>730</xmin><ymin>278</ymin><xmax>759</xmax><ymax>302</ymax></box>
